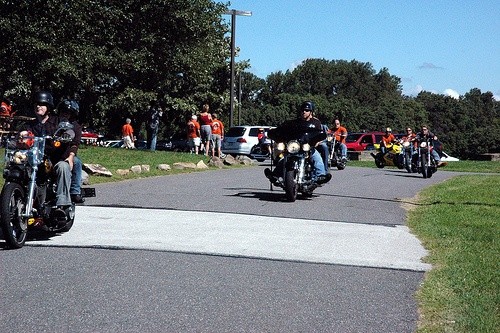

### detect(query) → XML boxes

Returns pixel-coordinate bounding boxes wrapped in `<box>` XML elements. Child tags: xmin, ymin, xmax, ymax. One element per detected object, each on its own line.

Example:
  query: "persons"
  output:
<box><xmin>379</xmin><ymin>125</ymin><xmax>449</xmax><ymax>167</ymax></box>
<box><xmin>185</xmin><ymin>103</ymin><xmax>225</xmax><ymax>159</ymax></box>
<box><xmin>257</xmin><ymin>100</ymin><xmax>347</xmax><ymax>187</ymax></box>
<box><xmin>121</xmin><ymin>118</ymin><xmax>137</xmax><ymax>150</ymax></box>
<box><xmin>0</xmin><ymin>89</ymin><xmax>86</xmax><ymax>218</ymax></box>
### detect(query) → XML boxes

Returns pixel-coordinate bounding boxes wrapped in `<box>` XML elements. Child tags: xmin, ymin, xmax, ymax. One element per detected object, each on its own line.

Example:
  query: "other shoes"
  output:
<box><xmin>265</xmin><ymin>168</ymin><xmax>276</xmax><ymax>184</ymax></box>
<box><xmin>72</xmin><ymin>194</ymin><xmax>85</xmax><ymax>203</ymax></box>
<box><xmin>55</xmin><ymin>207</ymin><xmax>66</xmax><ymax>217</ymax></box>
<box><xmin>318</xmin><ymin>173</ymin><xmax>332</xmax><ymax>184</ymax></box>
<box><xmin>341</xmin><ymin>156</ymin><xmax>347</xmax><ymax>162</ymax></box>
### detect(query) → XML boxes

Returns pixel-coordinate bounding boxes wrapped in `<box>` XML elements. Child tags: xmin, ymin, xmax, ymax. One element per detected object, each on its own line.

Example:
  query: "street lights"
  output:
<box><xmin>228</xmin><ymin>9</ymin><xmax>252</xmax><ymax>124</ymax></box>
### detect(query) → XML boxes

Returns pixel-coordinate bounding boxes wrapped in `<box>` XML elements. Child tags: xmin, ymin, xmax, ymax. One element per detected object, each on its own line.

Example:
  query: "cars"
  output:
<box><xmin>221</xmin><ymin>125</ymin><xmax>278</xmax><ymax>163</ymax></box>
<box><xmin>101</xmin><ymin>137</ymin><xmax>190</xmax><ymax>153</ymax></box>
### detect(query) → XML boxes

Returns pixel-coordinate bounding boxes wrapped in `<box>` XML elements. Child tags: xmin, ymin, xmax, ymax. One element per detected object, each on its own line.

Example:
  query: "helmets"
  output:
<box><xmin>300</xmin><ymin>101</ymin><xmax>312</xmax><ymax>112</ymax></box>
<box><xmin>31</xmin><ymin>91</ymin><xmax>54</xmax><ymax>107</ymax></box>
<box><xmin>58</xmin><ymin>100</ymin><xmax>79</xmax><ymax>116</ymax></box>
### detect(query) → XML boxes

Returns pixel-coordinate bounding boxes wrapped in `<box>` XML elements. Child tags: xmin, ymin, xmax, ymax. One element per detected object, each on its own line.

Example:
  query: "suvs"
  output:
<box><xmin>346</xmin><ymin>132</ymin><xmax>396</xmax><ymax>160</ymax></box>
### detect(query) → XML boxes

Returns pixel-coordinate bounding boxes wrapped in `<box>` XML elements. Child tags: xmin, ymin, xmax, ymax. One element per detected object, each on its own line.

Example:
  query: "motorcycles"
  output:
<box><xmin>268</xmin><ymin>127</ymin><xmax>326</xmax><ymax>202</ymax></box>
<box><xmin>370</xmin><ymin>135</ymin><xmax>448</xmax><ymax>179</ymax></box>
<box><xmin>327</xmin><ymin>131</ymin><xmax>349</xmax><ymax>170</ymax></box>
<box><xmin>0</xmin><ymin>120</ymin><xmax>85</xmax><ymax>249</ymax></box>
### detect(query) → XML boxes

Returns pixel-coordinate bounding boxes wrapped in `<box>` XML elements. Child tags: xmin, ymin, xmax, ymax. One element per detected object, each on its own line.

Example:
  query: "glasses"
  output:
<box><xmin>421</xmin><ymin>128</ymin><xmax>428</xmax><ymax>131</ymax></box>
<box><xmin>335</xmin><ymin>122</ymin><xmax>340</xmax><ymax>125</ymax></box>
<box><xmin>406</xmin><ymin>130</ymin><xmax>412</xmax><ymax>132</ymax></box>
